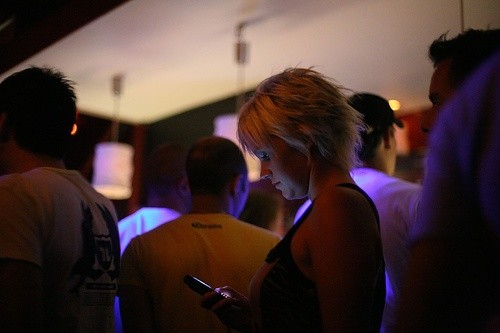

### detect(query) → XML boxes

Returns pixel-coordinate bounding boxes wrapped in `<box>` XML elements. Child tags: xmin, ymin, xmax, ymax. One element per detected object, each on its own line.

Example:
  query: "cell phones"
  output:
<box><xmin>184</xmin><ymin>274</ymin><xmax>228</xmax><ymax>305</ymax></box>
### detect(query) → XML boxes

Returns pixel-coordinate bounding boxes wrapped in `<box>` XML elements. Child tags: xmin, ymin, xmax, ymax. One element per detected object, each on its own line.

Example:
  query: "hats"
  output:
<box><xmin>348</xmin><ymin>93</ymin><xmax>403</xmax><ymax>128</ymax></box>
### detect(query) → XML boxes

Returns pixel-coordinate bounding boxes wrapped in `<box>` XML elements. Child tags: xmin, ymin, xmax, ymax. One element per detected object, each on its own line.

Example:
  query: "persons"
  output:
<box><xmin>294</xmin><ymin>92</ymin><xmax>422</xmax><ymax>333</ymax></box>
<box><xmin>405</xmin><ymin>22</ymin><xmax>500</xmax><ymax>333</ymax></box>
<box><xmin>200</xmin><ymin>60</ymin><xmax>387</xmax><ymax>333</ymax></box>
<box><xmin>0</xmin><ymin>65</ymin><xmax>123</xmax><ymax>333</ymax></box>
<box><xmin>114</xmin><ymin>135</ymin><xmax>283</xmax><ymax>333</ymax></box>
